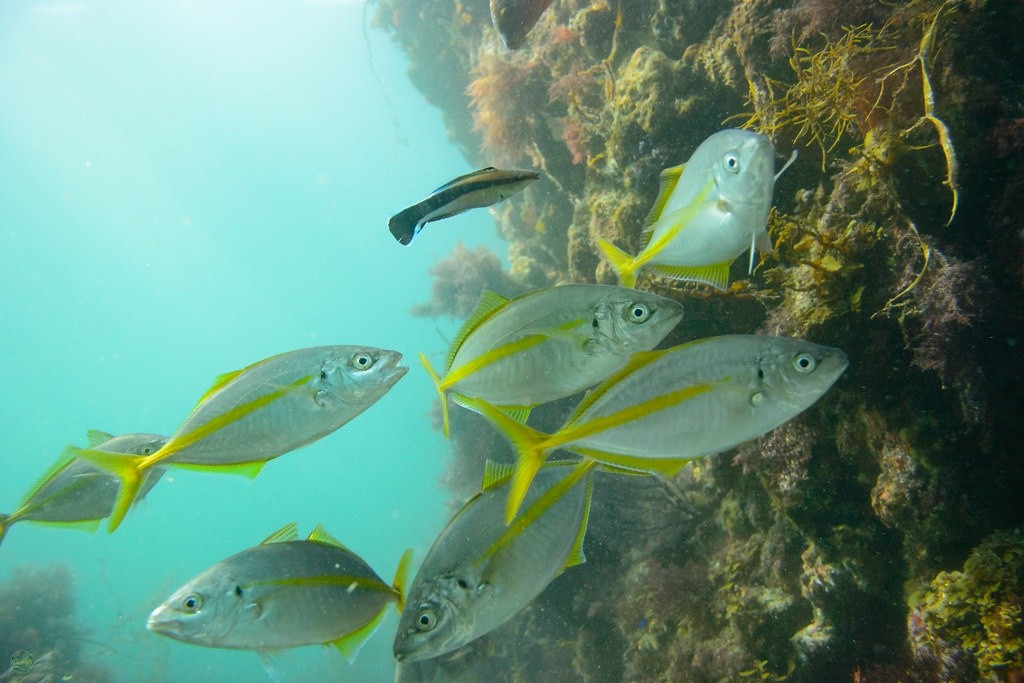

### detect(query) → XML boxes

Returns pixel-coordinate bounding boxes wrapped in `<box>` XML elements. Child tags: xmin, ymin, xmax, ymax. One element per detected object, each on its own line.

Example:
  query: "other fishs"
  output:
<box><xmin>0</xmin><ymin>130</ymin><xmax>851</xmax><ymax>662</ymax></box>
